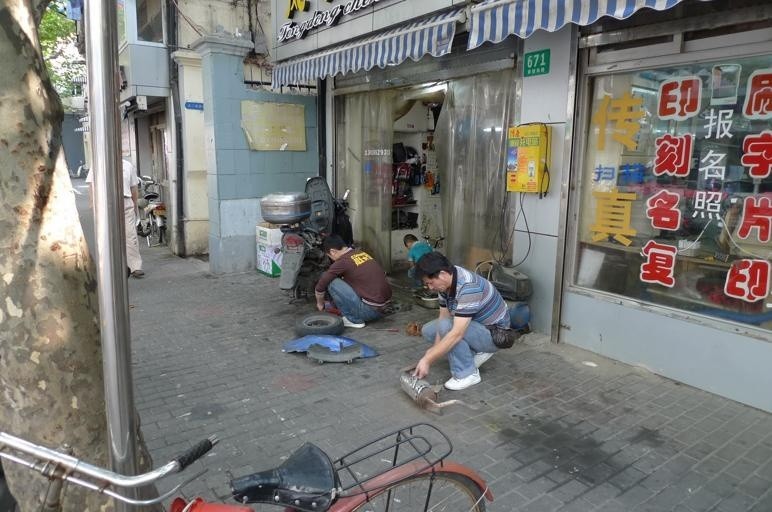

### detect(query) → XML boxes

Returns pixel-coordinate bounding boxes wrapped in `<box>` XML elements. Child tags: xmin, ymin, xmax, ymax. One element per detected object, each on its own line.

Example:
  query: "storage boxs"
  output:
<box><xmin>256</xmin><ymin>221</ymin><xmax>286</xmax><ymax>245</ymax></box>
<box><xmin>254</xmin><ymin>244</ymin><xmax>283</xmax><ymax>278</ymax></box>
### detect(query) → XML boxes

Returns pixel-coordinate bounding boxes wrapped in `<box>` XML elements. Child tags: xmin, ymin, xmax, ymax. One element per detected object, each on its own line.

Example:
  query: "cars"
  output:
<box><xmin>621</xmin><ymin>173</ymin><xmax>770</xmax><ymax>234</ymax></box>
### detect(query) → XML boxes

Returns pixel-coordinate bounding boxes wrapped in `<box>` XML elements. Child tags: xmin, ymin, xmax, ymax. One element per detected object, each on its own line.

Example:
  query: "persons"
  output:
<box><xmin>412</xmin><ymin>251</ymin><xmax>511</xmax><ymax>391</ymax></box>
<box><xmin>314</xmin><ymin>234</ymin><xmax>392</xmax><ymax>329</ymax></box>
<box><xmin>404</xmin><ymin>234</ymin><xmax>433</xmax><ymax>279</ymax></box>
<box><xmin>85</xmin><ymin>159</ymin><xmax>145</xmax><ymax>278</ymax></box>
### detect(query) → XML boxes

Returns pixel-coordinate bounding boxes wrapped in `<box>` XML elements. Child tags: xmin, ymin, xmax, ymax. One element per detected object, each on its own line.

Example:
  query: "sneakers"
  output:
<box><xmin>445</xmin><ymin>368</ymin><xmax>482</xmax><ymax>391</ymax></box>
<box><xmin>475</xmin><ymin>352</ymin><xmax>493</xmax><ymax>367</ymax></box>
<box><xmin>133</xmin><ymin>270</ymin><xmax>144</xmax><ymax>277</ymax></box>
<box><xmin>342</xmin><ymin>316</ymin><xmax>365</xmax><ymax>329</ymax></box>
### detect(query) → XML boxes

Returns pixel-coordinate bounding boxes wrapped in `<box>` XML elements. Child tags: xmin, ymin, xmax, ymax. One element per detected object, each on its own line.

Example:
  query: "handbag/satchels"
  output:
<box><xmin>489</xmin><ymin>324</ymin><xmax>516</xmax><ymax>348</ymax></box>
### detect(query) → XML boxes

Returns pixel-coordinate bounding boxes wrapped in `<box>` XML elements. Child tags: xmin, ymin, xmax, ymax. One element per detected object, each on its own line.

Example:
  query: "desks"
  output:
<box><xmin>573</xmin><ymin>234</ymin><xmax>772</xmax><ymax>331</ymax></box>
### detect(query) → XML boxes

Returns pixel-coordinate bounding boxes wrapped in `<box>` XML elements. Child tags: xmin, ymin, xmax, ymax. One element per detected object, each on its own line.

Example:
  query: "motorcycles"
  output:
<box><xmin>259</xmin><ymin>174</ymin><xmax>355</xmax><ymax>306</ymax></box>
<box><xmin>135</xmin><ymin>174</ymin><xmax>167</xmax><ymax>246</ymax></box>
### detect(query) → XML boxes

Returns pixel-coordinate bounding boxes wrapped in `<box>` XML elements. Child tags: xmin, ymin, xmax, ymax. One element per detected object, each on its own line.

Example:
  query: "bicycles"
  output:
<box><xmin>2</xmin><ymin>421</ymin><xmax>496</xmax><ymax>510</ymax></box>
